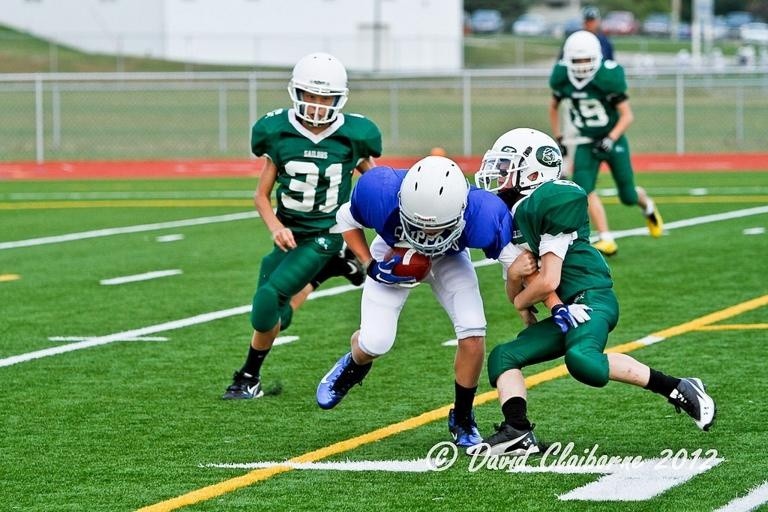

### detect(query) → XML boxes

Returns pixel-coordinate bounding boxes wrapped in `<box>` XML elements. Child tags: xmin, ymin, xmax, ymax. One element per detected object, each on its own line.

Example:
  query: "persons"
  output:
<box><xmin>547</xmin><ymin>29</ymin><xmax>663</xmax><ymax>255</ymax></box>
<box><xmin>556</xmin><ymin>6</ymin><xmax>613</xmax><ymax>65</ymax></box>
<box><xmin>315</xmin><ymin>155</ymin><xmax>593</xmax><ymax>448</ymax></box>
<box><xmin>465</xmin><ymin>126</ymin><xmax>716</xmax><ymax>456</ymax></box>
<box><xmin>217</xmin><ymin>51</ymin><xmax>382</xmax><ymax>401</ymax></box>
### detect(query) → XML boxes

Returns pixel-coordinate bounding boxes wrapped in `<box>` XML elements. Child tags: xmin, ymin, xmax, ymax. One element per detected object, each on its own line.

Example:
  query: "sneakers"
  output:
<box><xmin>591</xmin><ymin>239</ymin><xmax>617</xmax><ymax>255</ymax></box>
<box><xmin>467</xmin><ymin>423</ymin><xmax>542</xmax><ymax>455</ymax></box>
<box><xmin>643</xmin><ymin>203</ymin><xmax>663</xmax><ymax>237</ymax></box>
<box><xmin>222</xmin><ymin>371</ymin><xmax>265</xmax><ymax>400</ymax></box>
<box><xmin>449</xmin><ymin>407</ymin><xmax>483</xmax><ymax>447</ymax></box>
<box><xmin>339</xmin><ymin>241</ymin><xmax>365</xmax><ymax>286</ymax></box>
<box><xmin>666</xmin><ymin>378</ymin><xmax>716</xmax><ymax>430</ymax></box>
<box><xmin>317</xmin><ymin>353</ymin><xmax>373</xmax><ymax>409</ymax></box>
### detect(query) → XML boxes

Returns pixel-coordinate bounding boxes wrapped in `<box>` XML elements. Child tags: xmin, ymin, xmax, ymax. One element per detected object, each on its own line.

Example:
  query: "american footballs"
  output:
<box><xmin>384</xmin><ymin>248</ymin><xmax>430</xmax><ymax>281</ymax></box>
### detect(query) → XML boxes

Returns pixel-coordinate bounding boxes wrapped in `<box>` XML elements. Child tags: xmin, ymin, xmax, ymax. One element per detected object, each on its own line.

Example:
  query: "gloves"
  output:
<box><xmin>551</xmin><ymin>303</ymin><xmax>593</xmax><ymax>333</ymax></box>
<box><xmin>364</xmin><ymin>257</ymin><xmax>422</xmax><ymax>290</ymax></box>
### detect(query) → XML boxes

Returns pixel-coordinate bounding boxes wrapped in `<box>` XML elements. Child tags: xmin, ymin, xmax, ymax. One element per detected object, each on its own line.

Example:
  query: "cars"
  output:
<box><xmin>463</xmin><ymin>8</ymin><xmax>767</xmax><ymax>45</ymax></box>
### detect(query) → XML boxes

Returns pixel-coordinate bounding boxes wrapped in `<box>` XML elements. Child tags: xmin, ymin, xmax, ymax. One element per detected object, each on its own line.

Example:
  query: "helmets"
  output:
<box><xmin>561</xmin><ymin>32</ymin><xmax>601</xmax><ymax>80</ymax></box>
<box><xmin>287</xmin><ymin>54</ymin><xmax>350</xmax><ymax>126</ymax></box>
<box><xmin>400</xmin><ymin>155</ymin><xmax>468</xmax><ymax>257</ymax></box>
<box><xmin>476</xmin><ymin>128</ymin><xmax>565</xmax><ymax>192</ymax></box>
